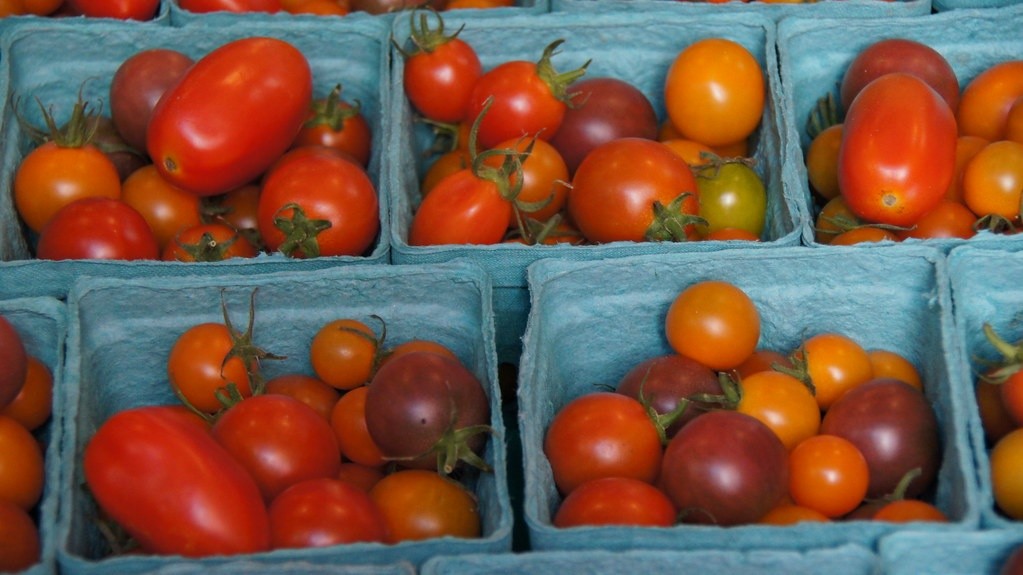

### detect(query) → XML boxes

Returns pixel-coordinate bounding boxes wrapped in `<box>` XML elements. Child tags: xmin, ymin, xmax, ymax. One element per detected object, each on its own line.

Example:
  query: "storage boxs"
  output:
<box><xmin>929</xmin><ymin>0</ymin><xmax>1023</xmax><ymax>18</ymax></box>
<box><xmin>168</xmin><ymin>0</ymin><xmax>553</xmax><ymax>23</ymax></box>
<box><xmin>2</xmin><ymin>21</ymin><xmax>392</xmax><ymax>299</ymax></box>
<box><xmin>0</xmin><ymin>293</ymin><xmax>68</xmax><ymax>575</ymax></box>
<box><xmin>946</xmin><ymin>245</ymin><xmax>1023</xmax><ymax>534</ymax></box>
<box><xmin>777</xmin><ymin>14</ymin><xmax>1021</xmax><ymax>250</ymax></box>
<box><xmin>549</xmin><ymin>0</ymin><xmax>935</xmax><ymax>20</ymax></box>
<box><xmin>0</xmin><ymin>0</ymin><xmax>168</xmax><ymax>28</ymax></box>
<box><xmin>63</xmin><ymin>254</ymin><xmax>515</xmax><ymax>565</ymax></box>
<box><xmin>30</xmin><ymin>556</ymin><xmax>420</xmax><ymax>575</ymax></box>
<box><xmin>872</xmin><ymin>531</ymin><xmax>1023</xmax><ymax>575</ymax></box>
<box><xmin>389</xmin><ymin>10</ymin><xmax>807</xmax><ymax>367</ymax></box>
<box><xmin>518</xmin><ymin>246</ymin><xmax>978</xmax><ymax>550</ymax></box>
<box><xmin>416</xmin><ymin>545</ymin><xmax>888</xmax><ymax>574</ymax></box>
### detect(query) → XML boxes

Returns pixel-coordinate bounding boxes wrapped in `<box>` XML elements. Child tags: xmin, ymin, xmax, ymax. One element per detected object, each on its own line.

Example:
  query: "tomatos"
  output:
<box><xmin>1</xmin><ymin>0</ymin><xmax>1023</xmax><ymax>575</ymax></box>
